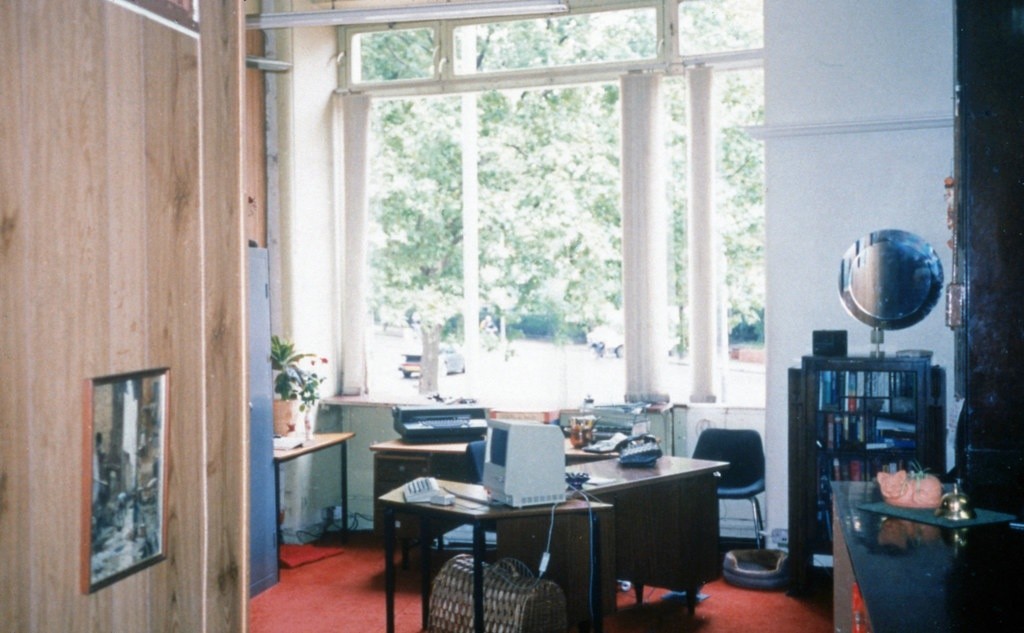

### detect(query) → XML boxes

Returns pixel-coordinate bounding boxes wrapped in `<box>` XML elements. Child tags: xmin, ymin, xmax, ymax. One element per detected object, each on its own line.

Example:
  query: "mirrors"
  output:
<box><xmin>837</xmin><ymin>229</ymin><xmax>944</xmax><ymax>331</ymax></box>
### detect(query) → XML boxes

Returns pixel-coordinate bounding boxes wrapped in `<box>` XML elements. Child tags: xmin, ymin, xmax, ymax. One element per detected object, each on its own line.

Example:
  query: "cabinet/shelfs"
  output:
<box><xmin>786</xmin><ymin>354</ymin><xmax>946</xmax><ymax>596</ymax></box>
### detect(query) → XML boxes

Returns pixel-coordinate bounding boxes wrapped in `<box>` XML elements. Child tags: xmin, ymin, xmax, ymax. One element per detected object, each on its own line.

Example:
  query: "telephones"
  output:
<box><xmin>615</xmin><ymin>434</ymin><xmax>664</xmax><ymax>469</ymax></box>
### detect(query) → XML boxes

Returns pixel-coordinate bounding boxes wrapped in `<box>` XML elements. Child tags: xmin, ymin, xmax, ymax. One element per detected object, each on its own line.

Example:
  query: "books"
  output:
<box><xmin>817</xmin><ymin>370</ymin><xmax>914</xmax><ymax>412</ymax></box>
<box><xmin>817</xmin><ymin>414</ymin><xmax>915</xmax><ymax>542</ymax></box>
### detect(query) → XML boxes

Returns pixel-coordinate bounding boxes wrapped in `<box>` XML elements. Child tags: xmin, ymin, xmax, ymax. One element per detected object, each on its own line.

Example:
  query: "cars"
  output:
<box><xmin>585</xmin><ymin>324</ymin><xmax>626</xmax><ymax>361</ymax></box>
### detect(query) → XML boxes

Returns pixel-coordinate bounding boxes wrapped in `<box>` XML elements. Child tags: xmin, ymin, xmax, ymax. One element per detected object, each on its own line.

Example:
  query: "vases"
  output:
<box><xmin>305</xmin><ymin>408</ymin><xmax>314</xmax><ymax>440</ymax></box>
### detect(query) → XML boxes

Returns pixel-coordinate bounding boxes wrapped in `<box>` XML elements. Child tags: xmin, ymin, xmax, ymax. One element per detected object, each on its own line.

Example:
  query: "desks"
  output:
<box><xmin>860</xmin><ymin>501</ymin><xmax>1018</xmax><ymax>633</ymax></box>
<box><xmin>272</xmin><ymin>431</ymin><xmax>355</xmax><ymax>582</ymax></box>
<box><xmin>370</xmin><ymin>437</ymin><xmax>730</xmax><ymax>633</ymax></box>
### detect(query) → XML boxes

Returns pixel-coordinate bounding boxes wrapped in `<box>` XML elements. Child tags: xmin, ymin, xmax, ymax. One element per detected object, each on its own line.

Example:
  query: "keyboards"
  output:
<box><xmin>403</xmin><ymin>477</ymin><xmax>439</xmax><ymax>502</ymax></box>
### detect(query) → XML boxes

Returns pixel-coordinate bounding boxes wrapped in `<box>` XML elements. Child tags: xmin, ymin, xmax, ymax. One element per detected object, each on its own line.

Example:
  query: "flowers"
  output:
<box><xmin>299</xmin><ymin>357</ymin><xmax>328</xmax><ymax>408</ymax></box>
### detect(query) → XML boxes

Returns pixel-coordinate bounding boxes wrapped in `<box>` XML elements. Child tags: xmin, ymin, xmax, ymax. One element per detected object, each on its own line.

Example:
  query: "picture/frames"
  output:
<box><xmin>80</xmin><ymin>366</ymin><xmax>171</xmax><ymax>596</ymax></box>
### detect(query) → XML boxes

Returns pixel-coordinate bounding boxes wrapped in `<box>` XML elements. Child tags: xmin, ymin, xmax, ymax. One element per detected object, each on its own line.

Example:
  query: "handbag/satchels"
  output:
<box><xmin>428</xmin><ymin>554</ymin><xmax>566</xmax><ymax>633</ymax></box>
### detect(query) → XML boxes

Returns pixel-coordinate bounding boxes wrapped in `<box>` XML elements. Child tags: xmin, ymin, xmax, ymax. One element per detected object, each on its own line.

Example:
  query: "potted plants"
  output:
<box><xmin>271</xmin><ymin>335</ymin><xmax>315</xmax><ymax>438</ymax></box>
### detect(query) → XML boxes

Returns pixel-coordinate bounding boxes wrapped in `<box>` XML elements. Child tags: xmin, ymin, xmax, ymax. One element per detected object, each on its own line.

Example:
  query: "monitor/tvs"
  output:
<box><xmin>483</xmin><ymin>419</ymin><xmax>566</xmax><ymax>507</ymax></box>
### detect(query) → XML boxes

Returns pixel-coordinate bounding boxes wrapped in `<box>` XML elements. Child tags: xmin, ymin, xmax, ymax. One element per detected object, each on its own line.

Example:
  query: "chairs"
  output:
<box><xmin>692</xmin><ymin>428</ymin><xmax>766</xmax><ymax>549</ymax></box>
